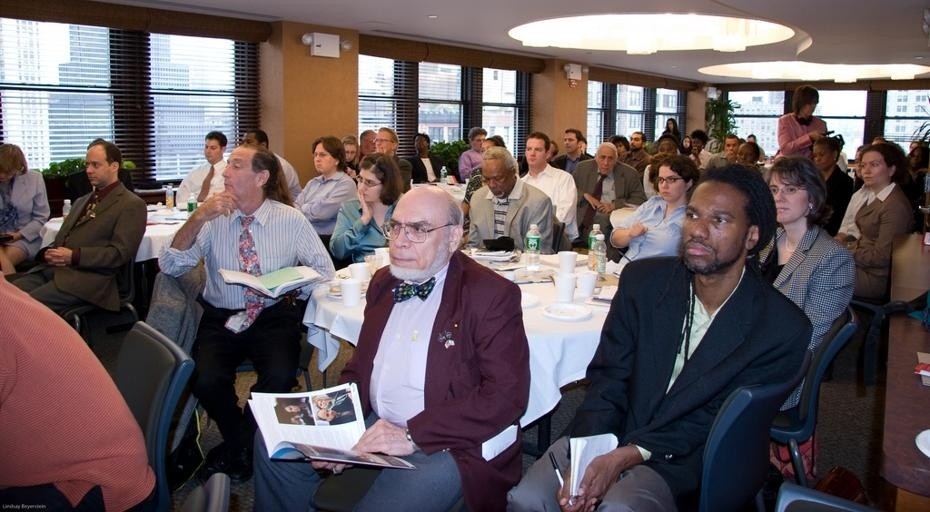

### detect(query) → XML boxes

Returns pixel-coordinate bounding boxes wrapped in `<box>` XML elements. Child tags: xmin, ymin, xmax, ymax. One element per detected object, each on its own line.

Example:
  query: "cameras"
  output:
<box><xmin>809</xmin><ymin>131</ymin><xmax>845</xmax><ymax>155</ymax></box>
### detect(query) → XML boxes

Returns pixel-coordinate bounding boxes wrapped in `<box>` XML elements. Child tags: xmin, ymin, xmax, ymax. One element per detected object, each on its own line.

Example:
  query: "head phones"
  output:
<box><xmin>793</xmin><ymin>114</ymin><xmax>813</xmax><ymax>125</ymax></box>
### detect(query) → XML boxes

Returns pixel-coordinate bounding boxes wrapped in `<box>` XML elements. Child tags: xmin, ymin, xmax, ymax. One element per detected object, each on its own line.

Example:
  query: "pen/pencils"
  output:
<box><xmin>549</xmin><ymin>450</ymin><xmax>572</xmax><ymax>506</ymax></box>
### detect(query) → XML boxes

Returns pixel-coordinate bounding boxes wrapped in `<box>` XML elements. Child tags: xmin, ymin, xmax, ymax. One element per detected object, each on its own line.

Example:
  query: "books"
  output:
<box><xmin>214</xmin><ymin>259</ymin><xmax>326</xmax><ymax>295</ymax></box>
<box><xmin>569</xmin><ymin>434</ymin><xmax>619</xmax><ymax>495</ymax></box>
<box><xmin>246</xmin><ymin>380</ymin><xmax>418</xmax><ymax>461</ymax></box>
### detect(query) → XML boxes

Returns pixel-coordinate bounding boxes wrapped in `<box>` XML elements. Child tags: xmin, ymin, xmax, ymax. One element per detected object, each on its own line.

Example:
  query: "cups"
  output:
<box><xmin>559</xmin><ymin>252</ymin><xmax>577</xmax><ymax>274</ymax></box>
<box><xmin>348</xmin><ymin>263</ymin><xmax>368</xmax><ymax>281</ymax></box>
<box><xmin>341</xmin><ymin>280</ymin><xmax>361</xmax><ymax>308</ymax></box>
<box><xmin>555</xmin><ymin>274</ymin><xmax>577</xmax><ymax>304</ymax></box>
<box><xmin>577</xmin><ymin>271</ymin><xmax>598</xmax><ymax>298</ymax></box>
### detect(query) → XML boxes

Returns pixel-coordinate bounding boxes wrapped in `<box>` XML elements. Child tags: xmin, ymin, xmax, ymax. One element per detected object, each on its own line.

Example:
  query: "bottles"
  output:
<box><xmin>523</xmin><ymin>223</ymin><xmax>542</xmax><ymax>273</ymax></box>
<box><xmin>187</xmin><ymin>192</ymin><xmax>198</xmax><ymax>215</ymax></box>
<box><xmin>592</xmin><ymin>234</ymin><xmax>607</xmax><ymax>273</ymax></box>
<box><xmin>63</xmin><ymin>199</ymin><xmax>72</xmax><ymax>216</ymax></box>
<box><xmin>440</xmin><ymin>165</ymin><xmax>447</xmax><ymax>185</ymax></box>
<box><xmin>165</xmin><ymin>184</ymin><xmax>174</xmax><ymax>210</ymax></box>
<box><xmin>587</xmin><ymin>225</ymin><xmax>602</xmax><ymax>271</ymax></box>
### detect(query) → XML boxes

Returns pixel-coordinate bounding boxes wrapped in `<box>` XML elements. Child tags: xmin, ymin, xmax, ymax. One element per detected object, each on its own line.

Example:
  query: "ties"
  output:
<box><xmin>197</xmin><ymin>165</ymin><xmax>215</xmax><ymax>203</ymax></box>
<box><xmin>223</xmin><ymin>215</ymin><xmax>262</xmax><ymax>335</ymax></box>
<box><xmin>581</xmin><ymin>172</ymin><xmax>608</xmax><ymax>234</ymax></box>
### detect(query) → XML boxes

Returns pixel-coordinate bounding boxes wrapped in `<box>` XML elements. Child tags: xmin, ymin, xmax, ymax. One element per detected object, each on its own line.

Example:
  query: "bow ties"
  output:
<box><xmin>391</xmin><ymin>276</ymin><xmax>435</xmax><ymax>303</ymax></box>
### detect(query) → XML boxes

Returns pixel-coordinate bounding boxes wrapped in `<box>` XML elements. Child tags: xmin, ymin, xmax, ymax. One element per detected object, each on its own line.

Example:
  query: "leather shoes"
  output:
<box><xmin>224</xmin><ymin>432</ymin><xmax>253</xmax><ymax>484</ymax></box>
<box><xmin>203</xmin><ymin>439</ymin><xmax>229</xmax><ymax>476</ymax></box>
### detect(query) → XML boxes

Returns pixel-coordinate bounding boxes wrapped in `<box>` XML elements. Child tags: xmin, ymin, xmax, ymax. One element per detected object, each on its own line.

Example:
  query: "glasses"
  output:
<box><xmin>480</xmin><ymin>173</ymin><xmax>508</xmax><ymax>184</ymax></box>
<box><xmin>379</xmin><ymin>220</ymin><xmax>455</xmax><ymax>243</ymax></box>
<box><xmin>372</xmin><ymin>138</ymin><xmax>396</xmax><ymax>144</ymax></box>
<box><xmin>355</xmin><ymin>175</ymin><xmax>381</xmax><ymax>187</ymax></box>
<box><xmin>656</xmin><ymin>176</ymin><xmax>684</xmax><ymax>183</ymax></box>
<box><xmin>768</xmin><ymin>185</ymin><xmax>807</xmax><ymax>196</ymax></box>
<box><xmin>311</xmin><ymin>151</ymin><xmax>328</xmax><ymax>157</ymax></box>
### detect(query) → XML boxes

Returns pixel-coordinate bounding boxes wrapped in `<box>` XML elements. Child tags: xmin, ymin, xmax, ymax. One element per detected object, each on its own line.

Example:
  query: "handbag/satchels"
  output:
<box><xmin>769</xmin><ymin>432</ymin><xmax>816</xmax><ymax>480</ymax></box>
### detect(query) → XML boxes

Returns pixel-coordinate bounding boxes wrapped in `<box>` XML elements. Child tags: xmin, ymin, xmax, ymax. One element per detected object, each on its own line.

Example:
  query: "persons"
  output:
<box><xmin>458</xmin><ymin>126</ymin><xmax>487</xmax><ymax>182</ymax></box>
<box><xmin>242</xmin><ymin>129</ymin><xmax>303</xmax><ymax>202</ymax></box>
<box><xmin>706</xmin><ymin>135</ymin><xmax>739</xmax><ymax>169</ymax></box>
<box><xmin>548</xmin><ymin>128</ymin><xmax>594</xmax><ymax>168</ymax></box>
<box><xmin>176</xmin><ymin>130</ymin><xmax>227</xmax><ymax>209</ymax></box>
<box><xmin>519</xmin><ymin>132</ymin><xmax>579</xmax><ymax>242</ymax></box>
<box><xmin>157</xmin><ymin>147</ymin><xmax>335</xmax><ymax>489</ymax></box>
<box><xmin>872</xmin><ymin>136</ymin><xmax>890</xmax><ymax>144</ymax></box>
<box><xmin>777</xmin><ymin>85</ymin><xmax>830</xmax><ymax>154</ymax></box>
<box><xmin>253</xmin><ymin>183</ymin><xmax>531</xmax><ymax>511</ymax></box>
<box><xmin>329</xmin><ymin>153</ymin><xmax>407</xmax><ymax>261</ymax></box>
<box><xmin>294</xmin><ymin>135</ymin><xmax>358</xmax><ymax>269</ymax></box>
<box><xmin>458</xmin><ymin>136</ymin><xmax>506</xmax><ymax>246</ymax></box>
<box><xmin>546</xmin><ymin>139</ymin><xmax>559</xmax><ymax>162</ymax></box>
<box><xmin>810</xmin><ymin>134</ymin><xmax>852</xmax><ymax>238</ymax></box>
<box><xmin>747</xmin><ymin>134</ymin><xmax>767</xmax><ymax>161</ymax></box>
<box><xmin>607</xmin><ymin>135</ymin><xmax>629</xmax><ymax>155</ymax></box>
<box><xmin>626</xmin><ymin>131</ymin><xmax>650</xmax><ymax>160</ymax></box>
<box><xmin>656</xmin><ymin>134</ymin><xmax>678</xmax><ymax>163</ymax></box>
<box><xmin>571</xmin><ymin>141</ymin><xmax>647</xmax><ymax>232</ymax></box>
<box><xmin>507</xmin><ymin>163</ymin><xmax>814</xmax><ymax>512</ymax></box>
<box><xmin>756</xmin><ymin>158</ymin><xmax>855</xmax><ymax>410</ymax></box>
<box><xmin>738</xmin><ymin>141</ymin><xmax>760</xmax><ymax>168</ymax></box>
<box><xmin>834</xmin><ymin>145</ymin><xmax>914</xmax><ymax>301</ymax></box>
<box><xmin>691</xmin><ymin>129</ymin><xmax>708</xmax><ymax>157</ymax></box>
<box><xmin>460</xmin><ymin>147</ymin><xmax>551</xmax><ymax>248</ymax></box>
<box><xmin>0</xmin><ymin>278</ymin><xmax>162</xmax><ymax>512</ymax></box>
<box><xmin>610</xmin><ymin>157</ymin><xmax>702</xmax><ymax>258</ymax></box>
<box><xmin>342</xmin><ymin>135</ymin><xmax>359</xmax><ymax>175</ymax></box>
<box><xmin>6</xmin><ymin>137</ymin><xmax>148</xmax><ymax>317</ymax></box>
<box><xmin>361</xmin><ymin>131</ymin><xmax>377</xmax><ymax>158</ymax></box>
<box><xmin>410</xmin><ymin>133</ymin><xmax>440</xmax><ymax>183</ymax></box>
<box><xmin>375</xmin><ymin>125</ymin><xmax>411</xmax><ymax>191</ymax></box>
<box><xmin>908</xmin><ymin>134</ymin><xmax>929</xmax><ymax>187</ymax></box>
<box><xmin>681</xmin><ymin>136</ymin><xmax>692</xmax><ymax>154</ymax></box>
<box><xmin>0</xmin><ymin>144</ymin><xmax>51</xmax><ymax>274</ymax></box>
<box><xmin>662</xmin><ymin>117</ymin><xmax>681</xmax><ymax>139</ymax></box>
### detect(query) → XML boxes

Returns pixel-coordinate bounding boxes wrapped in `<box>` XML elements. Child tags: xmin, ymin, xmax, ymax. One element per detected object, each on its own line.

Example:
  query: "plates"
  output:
<box><xmin>328</xmin><ymin>295</ymin><xmax>366</xmax><ymax>299</ymax></box>
<box><xmin>545</xmin><ymin>304</ymin><xmax>592</xmax><ymax>321</ymax></box>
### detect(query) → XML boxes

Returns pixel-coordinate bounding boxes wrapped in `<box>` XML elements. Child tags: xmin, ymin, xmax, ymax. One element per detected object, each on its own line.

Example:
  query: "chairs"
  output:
<box><xmin>850</xmin><ymin>282</ymin><xmax>904</xmax><ymax>371</ymax></box>
<box><xmin>766</xmin><ymin>304</ymin><xmax>860</xmax><ymax>487</ymax></box>
<box><xmin>696</xmin><ymin>344</ymin><xmax>817</xmax><ymax>511</ymax></box>
<box><xmin>109</xmin><ymin>318</ymin><xmax>198</xmax><ymax>510</ymax></box>
<box><xmin>60</xmin><ymin>255</ymin><xmax>141</xmax><ymax>335</ymax></box>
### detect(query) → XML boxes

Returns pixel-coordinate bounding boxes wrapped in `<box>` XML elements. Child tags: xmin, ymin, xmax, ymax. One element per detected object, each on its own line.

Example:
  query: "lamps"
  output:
<box><xmin>564</xmin><ymin>64</ymin><xmax>589</xmax><ymax>88</ymax></box>
<box><xmin>699</xmin><ymin>60</ymin><xmax>929</xmax><ymax>84</ymax></box>
<box><xmin>302</xmin><ymin>32</ymin><xmax>354</xmax><ymax>60</ymax></box>
<box><xmin>509</xmin><ymin>8</ymin><xmax>797</xmax><ymax>54</ymax></box>
